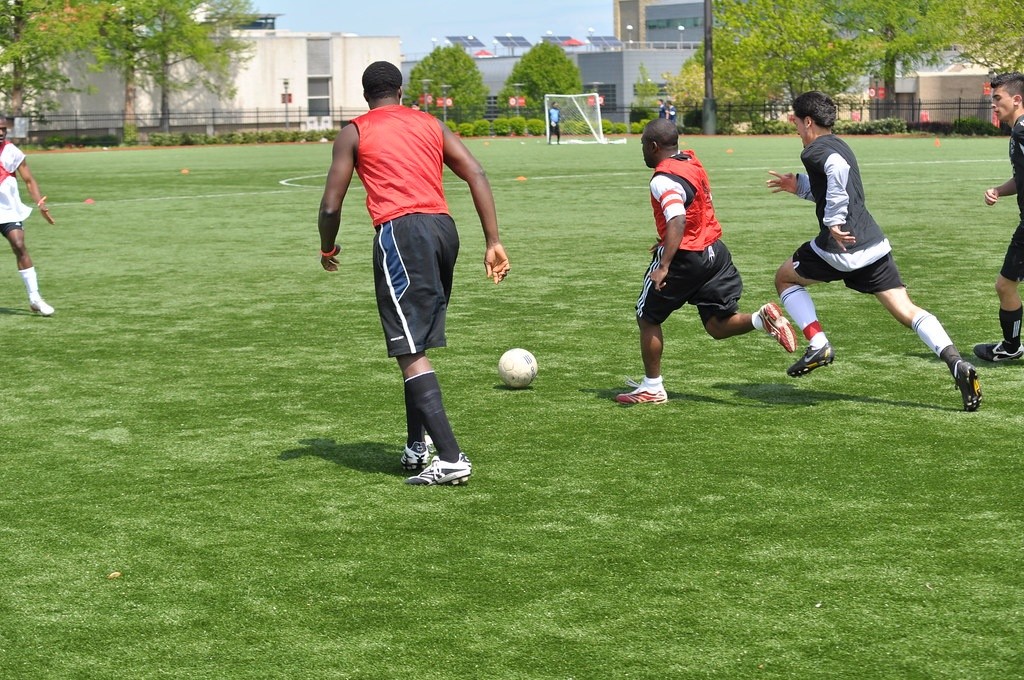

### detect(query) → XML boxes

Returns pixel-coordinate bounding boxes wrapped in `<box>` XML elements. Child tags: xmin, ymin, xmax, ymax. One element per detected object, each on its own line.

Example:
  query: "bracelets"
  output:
<box><xmin>37</xmin><ymin>196</ymin><xmax>47</xmax><ymax>206</ymax></box>
<box><xmin>320</xmin><ymin>245</ymin><xmax>336</xmax><ymax>256</ymax></box>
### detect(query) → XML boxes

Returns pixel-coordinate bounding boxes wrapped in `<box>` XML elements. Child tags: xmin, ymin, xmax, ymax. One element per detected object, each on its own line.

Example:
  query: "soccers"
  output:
<box><xmin>498</xmin><ymin>348</ymin><xmax>538</xmax><ymax>388</ymax></box>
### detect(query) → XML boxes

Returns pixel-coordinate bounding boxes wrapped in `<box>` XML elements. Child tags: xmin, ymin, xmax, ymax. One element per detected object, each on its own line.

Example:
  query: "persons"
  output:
<box><xmin>0</xmin><ymin>115</ymin><xmax>55</xmax><ymax>315</ymax></box>
<box><xmin>318</xmin><ymin>62</ymin><xmax>511</xmax><ymax>483</ymax></box>
<box><xmin>767</xmin><ymin>91</ymin><xmax>981</xmax><ymax>411</ymax></box>
<box><xmin>657</xmin><ymin>99</ymin><xmax>677</xmax><ymax>130</ymax></box>
<box><xmin>974</xmin><ymin>72</ymin><xmax>1024</xmax><ymax>363</ymax></box>
<box><xmin>615</xmin><ymin>119</ymin><xmax>796</xmax><ymax>407</ymax></box>
<box><xmin>548</xmin><ymin>102</ymin><xmax>560</xmax><ymax>145</ymax></box>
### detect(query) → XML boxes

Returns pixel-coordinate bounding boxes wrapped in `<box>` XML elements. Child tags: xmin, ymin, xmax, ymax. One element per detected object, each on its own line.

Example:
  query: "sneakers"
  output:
<box><xmin>974</xmin><ymin>341</ymin><xmax>1024</xmax><ymax>362</ymax></box>
<box><xmin>31</xmin><ymin>298</ymin><xmax>54</xmax><ymax>316</ymax></box>
<box><xmin>616</xmin><ymin>376</ymin><xmax>667</xmax><ymax>405</ymax></box>
<box><xmin>787</xmin><ymin>341</ymin><xmax>834</xmax><ymax>377</ymax></box>
<box><xmin>404</xmin><ymin>452</ymin><xmax>472</xmax><ymax>485</ymax></box>
<box><xmin>401</xmin><ymin>441</ymin><xmax>428</xmax><ymax>467</ymax></box>
<box><xmin>758</xmin><ymin>302</ymin><xmax>797</xmax><ymax>353</ymax></box>
<box><xmin>955</xmin><ymin>361</ymin><xmax>982</xmax><ymax>412</ymax></box>
